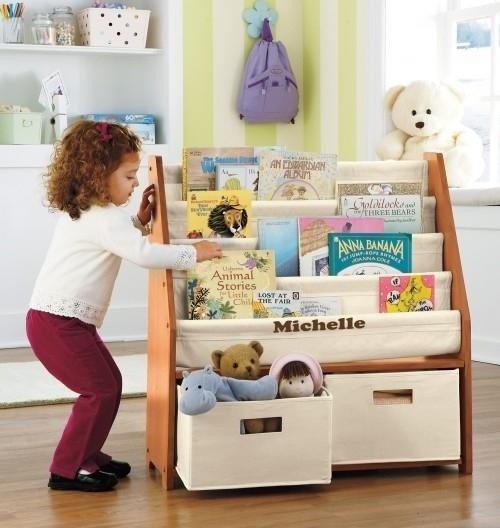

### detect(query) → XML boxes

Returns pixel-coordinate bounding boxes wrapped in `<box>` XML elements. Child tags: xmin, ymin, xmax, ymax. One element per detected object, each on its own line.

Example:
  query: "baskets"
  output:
<box><xmin>78</xmin><ymin>7</ymin><xmax>151</xmax><ymax>48</ymax></box>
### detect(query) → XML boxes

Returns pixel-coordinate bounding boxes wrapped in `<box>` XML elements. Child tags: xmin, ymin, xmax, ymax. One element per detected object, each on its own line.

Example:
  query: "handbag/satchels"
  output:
<box><xmin>238</xmin><ymin>19</ymin><xmax>299</xmax><ymax>125</ymax></box>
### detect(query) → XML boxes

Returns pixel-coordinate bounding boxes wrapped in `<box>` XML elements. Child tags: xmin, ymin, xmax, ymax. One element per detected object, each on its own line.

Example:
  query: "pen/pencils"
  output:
<box><xmin>0</xmin><ymin>0</ymin><xmax>26</xmax><ymax>17</ymax></box>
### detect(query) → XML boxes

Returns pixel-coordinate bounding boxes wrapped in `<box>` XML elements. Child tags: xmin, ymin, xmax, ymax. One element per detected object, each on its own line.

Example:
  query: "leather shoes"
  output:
<box><xmin>47</xmin><ymin>459</ymin><xmax>131</xmax><ymax>492</ymax></box>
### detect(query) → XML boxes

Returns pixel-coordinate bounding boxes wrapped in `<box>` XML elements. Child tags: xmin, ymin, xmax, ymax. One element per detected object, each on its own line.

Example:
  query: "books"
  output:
<box><xmin>182</xmin><ymin>146</ymin><xmax>437</xmax><ymax>321</ymax></box>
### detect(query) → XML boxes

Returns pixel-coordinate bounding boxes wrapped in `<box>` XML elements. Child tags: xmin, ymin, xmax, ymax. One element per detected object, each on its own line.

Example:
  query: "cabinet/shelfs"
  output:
<box><xmin>1</xmin><ymin>2</ymin><xmax>185</xmax><ymax>408</ymax></box>
<box><xmin>147</xmin><ymin>152</ymin><xmax>473</xmax><ymax>497</ymax></box>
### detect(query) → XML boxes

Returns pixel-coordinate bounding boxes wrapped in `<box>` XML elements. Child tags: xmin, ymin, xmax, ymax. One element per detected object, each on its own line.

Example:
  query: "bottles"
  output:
<box><xmin>29</xmin><ymin>6</ymin><xmax>78</xmax><ymax>45</ymax></box>
